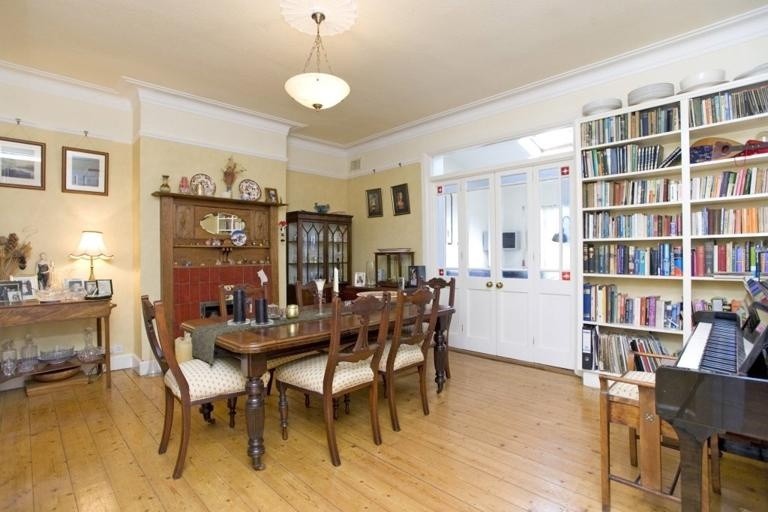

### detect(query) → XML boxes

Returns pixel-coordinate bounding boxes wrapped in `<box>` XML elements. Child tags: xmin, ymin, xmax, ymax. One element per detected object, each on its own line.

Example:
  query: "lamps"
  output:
<box><xmin>68</xmin><ymin>231</ymin><xmax>112</xmax><ymax>280</ymax></box>
<box><xmin>0</xmin><ymin>135</ymin><xmax>45</xmax><ymax>190</ymax></box>
<box><xmin>283</xmin><ymin>11</ymin><xmax>352</xmax><ymax>114</ymax></box>
<box><xmin>61</xmin><ymin>147</ymin><xmax>109</xmax><ymax>199</ymax></box>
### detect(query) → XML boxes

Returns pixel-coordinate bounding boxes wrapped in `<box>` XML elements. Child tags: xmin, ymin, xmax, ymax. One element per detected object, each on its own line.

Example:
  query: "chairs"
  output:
<box><xmin>275</xmin><ymin>292</ymin><xmax>392</xmax><ymax>468</ymax></box>
<box><xmin>344</xmin><ymin>282</ymin><xmax>440</xmax><ymax>432</ymax></box>
<box><xmin>295</xmin><ymin>276</ymin><xmax>337</xmax><ymax>307</ymax></box>
<box><xmin>140</xmin><ymin>294</ymin><xmax>272</xmax><ymax>481</ymax></box>
<box><xmin>419</xmin><ymin>277</ymin><xmax>454</xmax><ymax>379</ymax></box>
<box><xmin>217</xmin><ymin>284</ymin><xmax>265</xmax><ymax>410</ymax></box>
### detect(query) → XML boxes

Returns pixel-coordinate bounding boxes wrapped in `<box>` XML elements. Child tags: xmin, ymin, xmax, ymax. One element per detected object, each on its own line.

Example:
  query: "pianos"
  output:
<box><xmin>654</xmin><ymin>310</ymin><xmax>768</xmax><ymax>512</ymax></box>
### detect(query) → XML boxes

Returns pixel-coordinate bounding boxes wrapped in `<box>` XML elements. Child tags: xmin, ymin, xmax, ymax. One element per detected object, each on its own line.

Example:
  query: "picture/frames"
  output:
<box><xmin>69</xmin><ymin>282</ymin><xmax>84</xmax><ymax>302</ymax></box>
<box><xmin>390</xmin><ymin>183</ymin><xmax>411</xmax><ymax>216</ymax></box>
<box><xmin>84</xmin><ymin>281</ymin><xmax>95</xmax><ymax>298</ymax></box>
<box><xmin>97</xmin><ymin>280</ymin><xmax>112</xmax><ymax>297</ymax></box>
<box><xmin>0</xmin><ymin>280</ymin><xmax>23</xmax><ymax>302</ymax></box>
<box><xmin>10</xmin><ymin>274</ymin><xmax>40</xmax><ymax>304</ymax></box>
<box><xmin>355</xmin><ymin>272</ymin><xmax>367</xmax><ymax>287</ymax></box>
<box><xmin>366</xmin><ymin>188</ymin><xmax>382</xmax><ymax>218</ymax></box>
<box><xmin>8</xmin><ymin>291</ymin><xmax>23</xmax><ymax>304</ymax></box>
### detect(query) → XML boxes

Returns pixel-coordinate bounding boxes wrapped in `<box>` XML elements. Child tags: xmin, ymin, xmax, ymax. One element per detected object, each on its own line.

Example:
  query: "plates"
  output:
<box><xmin>355</xmin><ymin>290</ymin><xmax>407</xmax><ymax>301</ymax></box>
<box><xmin>265</xmin><ymin>314</ymin><xmax>281</xmax><ymax>318</ymax></box>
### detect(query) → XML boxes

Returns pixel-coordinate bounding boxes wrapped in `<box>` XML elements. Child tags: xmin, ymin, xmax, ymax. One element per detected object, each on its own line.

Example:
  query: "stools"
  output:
<box><xmin>598</xmin><ymin>353</ymin><xmax>721</xmax><ymax>511</ymax></box>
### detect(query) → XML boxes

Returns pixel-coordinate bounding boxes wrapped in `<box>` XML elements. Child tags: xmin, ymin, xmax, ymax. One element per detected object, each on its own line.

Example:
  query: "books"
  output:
<box><xmin>582</xmin><ymin>144</ymin><xmax>682</xmax><ymax>178</ymax></box>
<box><xmin>584</xmin><ymin>212</ymin><xmax>683</xmax><ymax>238</ymax></box>
<box><xmin>691</xmin><ymin>166</ymin><xmax>768</xmax><ymax>198</ymax></box>
<box><xmin>691</xmin><ymin>241</ymin><xmax>768</xmax><ymax>278</ymax></box>
<box><xmin>583</xmin><ymin>326</ymin><xmax>668</xmax><ymax>373</ymax></box>
<box><xmin>583</xmin><ymin>282</ymin><xmax>682</xmax><ymax>330</ymax></box>
<box><xmin>688</xmin><ymin>84</ymin><xmax>768</xmax><ymax>129</ymax></box>
<box><xmin>584</xmin><ymin>241</ymin><xmax>683</xmax><ymax>276</ymax></box>
<box><xmin>582</xmin><ymin>178</ymin><xmax>682</xmax><ymax>207</ymax></box>
<box><xmin>581</xmin><ymin>105</ymin><xmax>683</xmax><ymax>149</ymax></box>
<box><xmin>691</xmin><ymin>206</ymin><xmax>767</xmax><ymax>235</ymax></box>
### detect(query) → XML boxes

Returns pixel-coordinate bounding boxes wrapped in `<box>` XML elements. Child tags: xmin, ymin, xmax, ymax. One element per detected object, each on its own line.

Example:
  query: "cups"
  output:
<box><xmin>267</xmin><ymin>304</ymin><xmax>280</xmax><ymax>316</ymax></box>
<box><xmin>285</xmin><ymin>305</ymin><xmax>299</xmax><ymax>319</ymax></box>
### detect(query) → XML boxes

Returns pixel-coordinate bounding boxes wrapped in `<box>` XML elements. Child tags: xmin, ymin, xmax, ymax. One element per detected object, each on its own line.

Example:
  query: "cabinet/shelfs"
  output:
<box><xmin>153</xmin><ymin>192</ymin><xmax>288</xmax><ymax>357</ymax></box>
<box><xmin>376</xmin><ymin>252</ymin><xmax>414</xmax><ymax>288</ymax></box>
<box><xmin>687</xmin><ymin>73</ymin><xmax>768</xmax><ymax>345</ymax></box>
<box><xmin>287</xmin><ymin>211</ymin><xmax>353</xmax><ymax>304</ymax></box>
<box><xmin>575</xmin><ymin>95</ymin><xmax>684</xmax><ymax>388</ymax></box>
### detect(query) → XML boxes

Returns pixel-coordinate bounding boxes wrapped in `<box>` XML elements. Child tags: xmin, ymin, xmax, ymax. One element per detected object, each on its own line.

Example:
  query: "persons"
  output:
<box><xmin>35</xmin><ymin>252</ymin><xmax>54</xmax><ymax>291</ymax></box>
